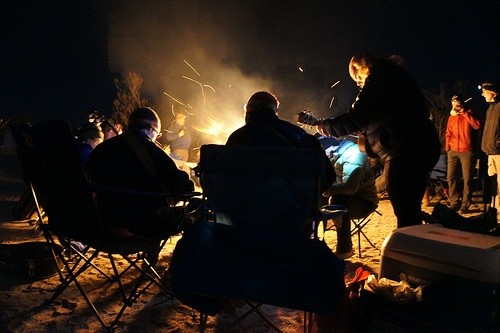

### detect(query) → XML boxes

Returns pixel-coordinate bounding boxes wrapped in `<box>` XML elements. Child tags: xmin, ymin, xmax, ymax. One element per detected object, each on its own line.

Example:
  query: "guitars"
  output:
<box><xmin>297</xmin><ymin>109</ymin><xmax>380</xmax><ymax>158</ymax></box>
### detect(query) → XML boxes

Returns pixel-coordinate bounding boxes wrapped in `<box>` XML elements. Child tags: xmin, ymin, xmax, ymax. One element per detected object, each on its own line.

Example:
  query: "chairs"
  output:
<box><xmin>8</xmin><ymin>116</ymin><xmax>494</xmax><ymax>333</ymax></box>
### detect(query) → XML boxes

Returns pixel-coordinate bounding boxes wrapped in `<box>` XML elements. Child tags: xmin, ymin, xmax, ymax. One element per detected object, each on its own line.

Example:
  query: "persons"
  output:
<box><xmin>445</xmin><ymin>95</ymin><xmax>482</xmax><ymax>213</ymax></box>
<box><xmin>478</xmin><ymin>78</ymin><xmax>500</xmax><ymax>227</ymax></box>
<box><xmin>86</xmin><ymin>108</ymin><xmax>205</xmax><ymax>272</ymax></box>
<box><xmin>310</xmin><ymin>123</ymin><xmax>379</xmax><ymax>260</ymax></box>
<box><xmin>41</xmin><ymin>122</ymin><xmax>114</xmax><ymax>254</ymax></box>
<box><xmin>200</xmin><ymin>92</ymin><xmax>346</xmax><ymax>333</ymax></box>
<box><xmin>296</xmin><ymin>54</ymin><xmax>441</xmax><ymax>229</ymax></box>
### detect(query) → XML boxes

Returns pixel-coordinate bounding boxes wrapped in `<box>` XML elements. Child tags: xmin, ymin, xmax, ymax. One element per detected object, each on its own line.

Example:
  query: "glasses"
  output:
<box><xmin>147</xmin><ymin>126</ymin><xmax>163</xmax><ymax>138</ymax></box>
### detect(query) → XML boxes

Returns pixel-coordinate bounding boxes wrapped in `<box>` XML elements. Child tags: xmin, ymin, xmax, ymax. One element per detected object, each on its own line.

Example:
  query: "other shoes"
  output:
<box><xmin>447</xmin><ymin>204</ymin><xmax>460</xmax><ymax>210</ymax></box>
<box><xmin>142</xmin><ymin>256</ymin><xmax>159</xmax><ymax>270</ymax></box>
<box><xmin>335</xmin><ymin>250</ymin><xmax>353</xmax><ymax>259</ymax></box>
<box><xmin>458</xmin><ymin>205</ymin><xmax>468</xmax><ymax>214</ymax></box>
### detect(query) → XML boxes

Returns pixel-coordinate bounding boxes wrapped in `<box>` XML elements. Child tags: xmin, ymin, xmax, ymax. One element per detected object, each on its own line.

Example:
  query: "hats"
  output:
<box><xmin>482</xmin><ymin>81</ymin><xmax>500</xmax><ymax>94</ymax></box>
